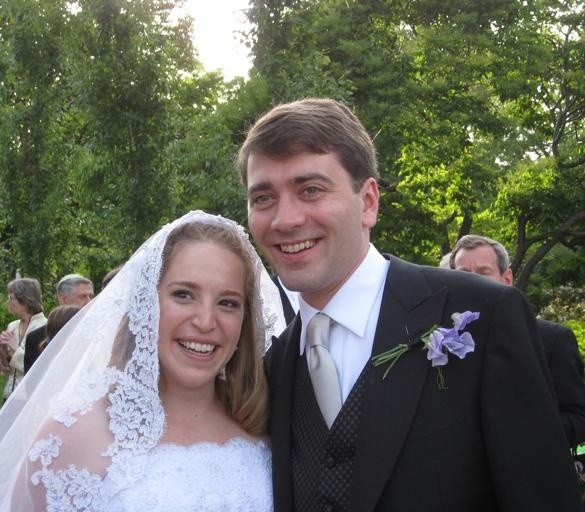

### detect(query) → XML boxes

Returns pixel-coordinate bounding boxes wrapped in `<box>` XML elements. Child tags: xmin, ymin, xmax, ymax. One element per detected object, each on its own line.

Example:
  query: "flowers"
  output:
<box><xmin>370</xmin><ymin>308</ymin><xmax>481</xmax><ymax>379</ymax></box>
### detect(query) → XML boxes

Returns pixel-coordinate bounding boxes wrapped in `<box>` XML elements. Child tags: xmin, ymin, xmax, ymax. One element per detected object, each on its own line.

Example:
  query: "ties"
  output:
<box><xmin>307</xmin><ymin>314</ymin><xmax>343</xmax><ymax>430</ymax></box>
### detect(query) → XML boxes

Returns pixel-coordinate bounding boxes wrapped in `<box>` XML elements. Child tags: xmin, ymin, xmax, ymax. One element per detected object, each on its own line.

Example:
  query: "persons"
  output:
<box><xmin>238</xmin><ymin>98</ymin><xmax>585</xmax><ymax>512</ymax></box>
<box><xmin>438</xmin><ymin>234</ymin><xmax>584</xmax><ymax>479</ymax></box>
<box><xmin>1</xmin><ymin>209</ymin><xmax>288</xmax><ymax>511</ymax></box>
<box><xmin>1</xmin><ymin>267</ymin><xmax>124</xmax><ymax>405</ymax></box>
